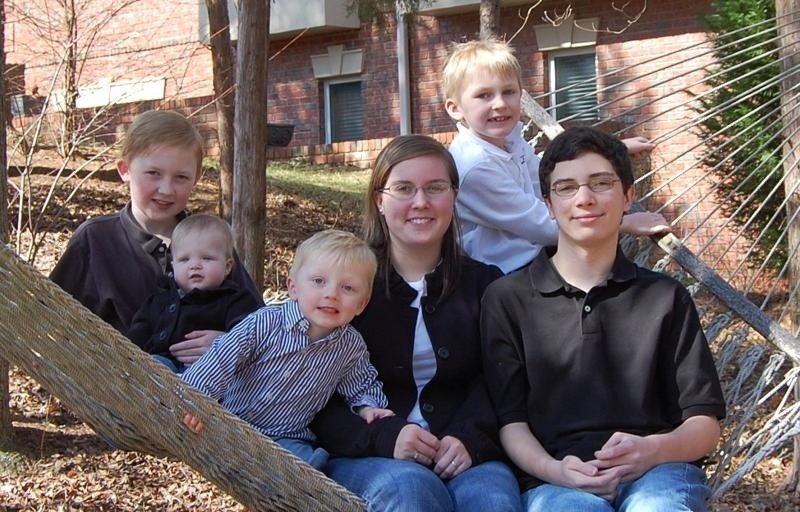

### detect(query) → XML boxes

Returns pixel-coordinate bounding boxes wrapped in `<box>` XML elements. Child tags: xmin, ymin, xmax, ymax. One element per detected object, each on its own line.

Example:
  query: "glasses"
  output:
<box><xmin>546</xmin><ymin>176</ymin><xmax>622</xmax><ymax>198</ymax></box>
<box><xmin>376</xmin><ymin>181</ymin><xmax>458</xmax><ymax>200</ymax></box>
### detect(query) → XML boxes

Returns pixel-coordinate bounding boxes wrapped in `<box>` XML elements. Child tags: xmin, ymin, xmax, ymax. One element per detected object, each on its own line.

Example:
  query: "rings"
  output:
<box><xmin>410</xmin><ymin>451</ymin><xmax>421</xmax><ymax>457</ymax></box>
<box><xmin>450</xmin><ymin>460</ymin><xmax>457</xmax><ymax>467</ymax></box>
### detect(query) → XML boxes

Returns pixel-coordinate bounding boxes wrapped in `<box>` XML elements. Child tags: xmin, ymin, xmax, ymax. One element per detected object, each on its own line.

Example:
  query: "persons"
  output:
<box><xmin>181</xmin><ymin>229</ymin><xmax>396</xmax><ymax>467</ymax></box>
<box><xmin>305</xmin><ymin>136</ymin><xmax>525</xmax><ymax>510</ymax></box>
<box><xmin>478</xmin><ymin>128</ymin><xmax>727</xmax><ymax>511</ymax></box>
<box><xmin>122</xmin><ymin>213</ymin><xmax>261</xmax><ymax>376</ymax></box>
<box><xmin>48</xmin><ymin>108</ymin><xmax>266</xmax><ymax>310</ymax></box>
<box><xmin>442</xmin><ymin>35</ymin><xmax>671</xmax><ymax>274</ymax></box>
